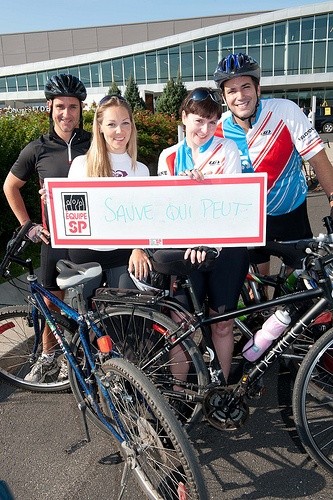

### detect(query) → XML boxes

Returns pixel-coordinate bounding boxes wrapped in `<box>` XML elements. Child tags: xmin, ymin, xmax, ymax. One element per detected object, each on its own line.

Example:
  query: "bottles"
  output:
<box><xmin>237</xmin><ymin>299</ymin><xmax>252</xmax><ymax>326</ymax></box>
<box><xmin>283</xmin><ymin>270</ymin><xmax>304</xmax><ymax>292</ymax></box>
<box><xmin>242</xmin><ymin>309</ymin><xmax>291</xmax><ymax>362</ymax></box>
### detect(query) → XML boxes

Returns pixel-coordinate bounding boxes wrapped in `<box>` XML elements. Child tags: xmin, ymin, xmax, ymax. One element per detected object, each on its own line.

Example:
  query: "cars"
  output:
<box><xmin>302</xmin><ymin>105</ymin><xmax>333</xmax><ymax>134</ymax></box>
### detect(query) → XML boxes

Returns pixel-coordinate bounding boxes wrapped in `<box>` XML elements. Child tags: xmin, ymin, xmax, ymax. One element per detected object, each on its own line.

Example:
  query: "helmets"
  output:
<box><xmin>214</xmin><ymin>53</ymin><xmax>260</xmax><ymax>88</ymax></box>
<box><xmin>44</xmin><ymin>73</ymin><xmax>87</xmax><ymax>100</ymax></box>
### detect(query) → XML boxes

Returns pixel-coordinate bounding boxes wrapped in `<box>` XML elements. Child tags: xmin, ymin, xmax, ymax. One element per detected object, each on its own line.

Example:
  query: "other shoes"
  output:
<box><xmin>168</xmin><ymin>397</ymin><xmax>184</xmax><ymax>427</ymax></box>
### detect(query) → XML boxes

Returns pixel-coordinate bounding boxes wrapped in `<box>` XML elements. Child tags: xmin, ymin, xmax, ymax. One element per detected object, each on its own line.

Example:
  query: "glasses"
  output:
<box><xmin>186</xmin><ymin>89</ymin><xmax>224</xmax><ymax>107</ymax></box>
<box><xmin>99</xmin><ymin>93</ymin><xmax>126</xmax><ymax>107</ymax></box>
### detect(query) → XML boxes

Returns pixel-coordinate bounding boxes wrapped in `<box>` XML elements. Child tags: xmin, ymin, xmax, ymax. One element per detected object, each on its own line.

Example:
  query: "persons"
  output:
<box><xmin>3</xmin><ymin>73</ymin><xmax>92</xmax><ymax>384</ymax></box>
<box><xmin>159</xmin><ymin>87</ymin><xmax>241</xmax><ymax>418</ymax></box>
<box><xmin>68</xmin><ymin>95</ymin><xmax>152</xmax><ymax>367</ymax></box>
<box><xmin>213</xmin><ymin>53</ymin><xmax>333</xmax><ymax>311</ymax></box>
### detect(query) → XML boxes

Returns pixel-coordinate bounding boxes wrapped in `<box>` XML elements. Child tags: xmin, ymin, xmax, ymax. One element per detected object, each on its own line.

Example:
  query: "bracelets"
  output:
<box><xmin>328</xmin><ymin>193</ymin><xmax>333</xmax><ymax>203</ymax></box>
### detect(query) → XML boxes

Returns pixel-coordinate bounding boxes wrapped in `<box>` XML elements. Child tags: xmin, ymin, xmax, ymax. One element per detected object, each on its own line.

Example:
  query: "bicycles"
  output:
<box><xmin>0</xmin><ymin>221</ymin><xmax>212</xmax><ymax>500</ymax></box>
<box><xmin>65</xmin><ymin>208</ymin><xmax>333</xmax><ymax>481</ymax></box>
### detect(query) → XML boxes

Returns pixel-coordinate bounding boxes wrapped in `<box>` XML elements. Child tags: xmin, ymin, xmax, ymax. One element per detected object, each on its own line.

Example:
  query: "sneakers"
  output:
<box><xmin>22</xmin><ymin>356</ymin><xmax>59</xmax><ymax>382</ymax></box>
<box><xmin>55</xmin><ymin>357</ymin><xmax>68</xmax><ymax>380</ymax></box>
